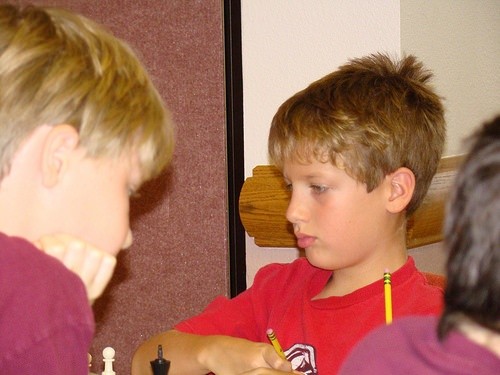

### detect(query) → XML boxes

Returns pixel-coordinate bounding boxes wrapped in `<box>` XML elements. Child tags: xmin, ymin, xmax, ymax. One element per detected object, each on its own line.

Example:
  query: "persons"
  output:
<box><xmin>0</xmin><ymin>0</ymin><xmax>176</xmax><ymax>375</ymax></box>
<box><xmin>131</xmin><ymin>50</ymin><xmax>446</xmax><ymax>375</ymax></box>
<box><xmin>339</xmin><ymin>113</ymin><xmax>500</xmax><ymax>375</ymax></box>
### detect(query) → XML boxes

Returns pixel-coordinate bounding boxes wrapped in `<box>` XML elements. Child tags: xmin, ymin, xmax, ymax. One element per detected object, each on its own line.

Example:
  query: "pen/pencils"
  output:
<box><xmin>265</xmin><ymin>328</ymin><xmax>289</xmax><ymax>364</ymax></box>
<box><xmin>383</xmin><ymin>267</ymin><xmax>393</xmax><ymax>325</ymax></box>
